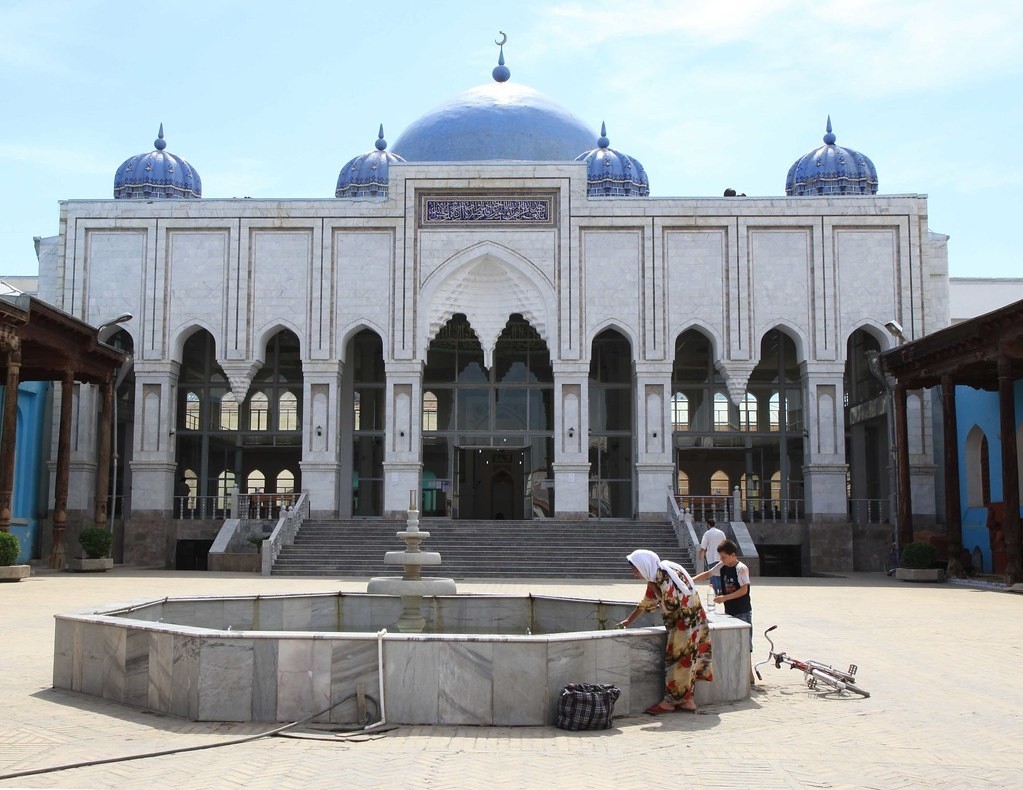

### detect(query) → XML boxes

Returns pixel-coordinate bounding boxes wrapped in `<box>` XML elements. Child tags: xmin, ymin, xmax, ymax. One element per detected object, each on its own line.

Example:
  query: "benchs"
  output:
<box><xmin>673</xmin><ymin>494</ymin><xmax>730</xmax><ymax>521</ymax></box>
<box><xmin>251</xmin><ymin>492</ymin><xmax>293</xmax><ymax>518</ymax></box>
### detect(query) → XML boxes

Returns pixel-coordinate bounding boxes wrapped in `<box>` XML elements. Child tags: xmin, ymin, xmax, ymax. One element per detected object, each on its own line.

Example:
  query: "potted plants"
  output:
<box><xmin>69</xmin><ymin>527</ymin><xmax>114</xmax><ymax>571</ymax></box>
<box><xmin>0</xmin><ymin>531</ymin><xmax>30</xmax><ymax>582</ymax></box>
<box><xmin>896</xmin><ymin>541</ymin><xmax>943</xmax><ymax>582</ymax></box>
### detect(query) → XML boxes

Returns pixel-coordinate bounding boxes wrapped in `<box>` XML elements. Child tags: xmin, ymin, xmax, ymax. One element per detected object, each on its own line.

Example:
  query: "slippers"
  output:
<box><xmin>645</xmin><ymin>703</ymin><xmax>673</xmax><ymax>714</ymax></box>
<box><xmin>672</xmin><ymin>704</ymin><xmax>695</xmax><ymax>714</ymax></box>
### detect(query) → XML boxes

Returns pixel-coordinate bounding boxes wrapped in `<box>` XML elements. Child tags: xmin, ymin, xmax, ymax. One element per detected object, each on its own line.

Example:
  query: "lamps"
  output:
<box><xmin>885</xmin><ymin>321</ymin><xmax>908</xmax><ymax>344</ymax></box>
<box><xmin>317</xmin><ymin>426</ymin><xmax>322</xmax><ymax>436</ymax></box>
<box><xmin>569</xmin><ymin>427</ymin><xmax>574</xmax><ymax>437</ymax></box>
<box><xmin>169</xmin><ymin>427</ymin><xmax>176</xmax><ymax>436</ymax></box>
<box><xmin>803</xmin><ymin>428</ymin><xmax>808</xmax><ymax>438</ymax></box>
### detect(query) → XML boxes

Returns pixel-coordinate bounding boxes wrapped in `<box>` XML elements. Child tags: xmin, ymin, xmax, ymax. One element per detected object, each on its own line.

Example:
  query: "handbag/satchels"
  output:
<box><xmin>554</xmin><ymin>683</ymin><xmax>621</xmax><ymax>731</ymax></box>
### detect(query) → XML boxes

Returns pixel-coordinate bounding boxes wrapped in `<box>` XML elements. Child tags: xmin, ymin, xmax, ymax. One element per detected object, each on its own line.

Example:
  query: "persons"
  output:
<box><xmin>613</xmin><ymin>549</ymin><xmax>713</xmax><ymax>715</ymax></box>
<box><xmin>691</xmin><ymin>539</ymin><xmax>754</xmax><ymax>684</ymax></box>
<box><xmin>699</xmin><ymin>518</ymin><xmax>727</xmax><ymax>597</ymax></box>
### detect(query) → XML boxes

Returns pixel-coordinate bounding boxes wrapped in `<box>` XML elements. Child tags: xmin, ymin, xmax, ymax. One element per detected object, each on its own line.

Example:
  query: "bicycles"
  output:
<box><xmin>755</xmin><ymin>625</ymin><xmax>871</xmax><ymax>698</ymax></box>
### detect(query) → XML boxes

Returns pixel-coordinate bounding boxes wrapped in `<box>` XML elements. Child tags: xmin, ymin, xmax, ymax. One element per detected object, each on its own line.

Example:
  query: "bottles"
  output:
<box><xmin>707</xmin><ymin>584</ymin><xmax>715</xmax><ymax>612</ymax></box>
